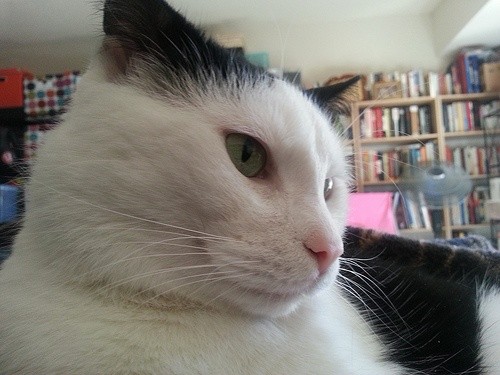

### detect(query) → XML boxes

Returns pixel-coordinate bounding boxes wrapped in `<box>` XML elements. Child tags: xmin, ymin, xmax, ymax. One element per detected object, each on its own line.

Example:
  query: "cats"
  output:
<box><xmin>1</xmin><ymin>0</ymin><xmax>499</xmax><ymax>375</ymax></box>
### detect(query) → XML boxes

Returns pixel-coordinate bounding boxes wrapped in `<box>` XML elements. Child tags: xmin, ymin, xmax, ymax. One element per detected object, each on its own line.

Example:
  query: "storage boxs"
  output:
<box><xmin>0</xmin><ymin>68</ymin><xmax>34</xmax><ymax>107</ymax></box>
<box><xmin>23</xmin><ymin>72</ymin><xmax>80</xmax><ymax>165</ymax></box>
<box><xmin>479</xmin><ymin>62</ymin><xmax>500</xmax><ymax>91</ymax></box>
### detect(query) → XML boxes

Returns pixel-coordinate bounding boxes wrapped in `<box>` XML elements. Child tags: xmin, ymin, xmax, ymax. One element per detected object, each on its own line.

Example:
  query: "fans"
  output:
<box><xmin>398</xmin><ymin>159</ymin><xmax>474</xmax><ymax>240</ymax></box>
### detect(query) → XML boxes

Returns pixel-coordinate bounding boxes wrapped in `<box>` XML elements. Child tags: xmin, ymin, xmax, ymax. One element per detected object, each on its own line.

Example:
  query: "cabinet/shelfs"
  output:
<box><xmin>335</xmin><ymin>92</ymin><xmax>500</xmax><ymax>241</ymax></box>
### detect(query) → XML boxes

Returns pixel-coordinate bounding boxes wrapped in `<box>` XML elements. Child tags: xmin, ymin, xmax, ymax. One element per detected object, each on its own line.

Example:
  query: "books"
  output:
<box><xmin>329</xmin><ymin>46</ymin><xmax>500</xmax><ymax>251</ymax></box>
